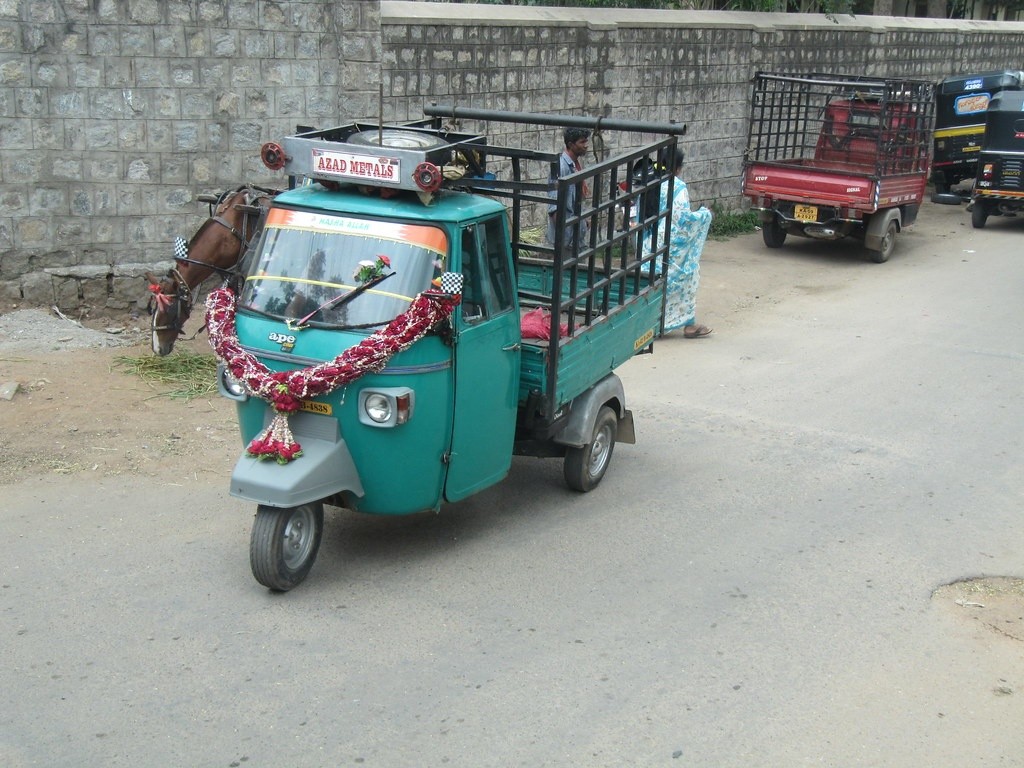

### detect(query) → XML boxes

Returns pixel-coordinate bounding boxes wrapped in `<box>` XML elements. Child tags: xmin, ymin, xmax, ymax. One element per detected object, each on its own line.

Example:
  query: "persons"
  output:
<box><xmin>545</xmin><ymin>127</ymin><xmax>591</xmax><ymax>263</ymax></box>
<box><xmin>637</xmin><ymin>147</ymin><xmax>715</xmax><ymax>338</ymax></box>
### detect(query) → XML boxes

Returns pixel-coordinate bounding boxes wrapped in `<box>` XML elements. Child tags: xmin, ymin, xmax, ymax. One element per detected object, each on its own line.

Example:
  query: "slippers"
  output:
<box><xmin>684</xmin><ymin>325</ymin><xmax>713</xmax><ymax>337</ymax></box>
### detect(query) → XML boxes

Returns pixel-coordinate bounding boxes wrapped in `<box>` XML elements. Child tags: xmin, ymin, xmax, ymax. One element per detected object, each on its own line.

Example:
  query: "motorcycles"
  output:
<box><xmin>742</xmin><ymin>70</ymin><xmax>936</xmax><ymax>263</ymax></box>
<box><xmin>933</xmin><ymin>70</ymin><xmax>1024</xmax><ymax>195</ymax></box>
<box><xmin>971</xmin><ymin>90</ymin><xmax>1024</xmax><ymax>229</ymax></box>
<box><xmin>203</xmin><ymin>102</ymin><xmax>688</xmax><ymax>591</ymax></box>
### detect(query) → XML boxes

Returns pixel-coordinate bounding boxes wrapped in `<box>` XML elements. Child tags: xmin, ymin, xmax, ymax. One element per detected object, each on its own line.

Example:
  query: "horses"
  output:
<box><xmin>143</xmin><ymin>181</ymin><xmax>263</xmax><ymax>357</ymax></box>
